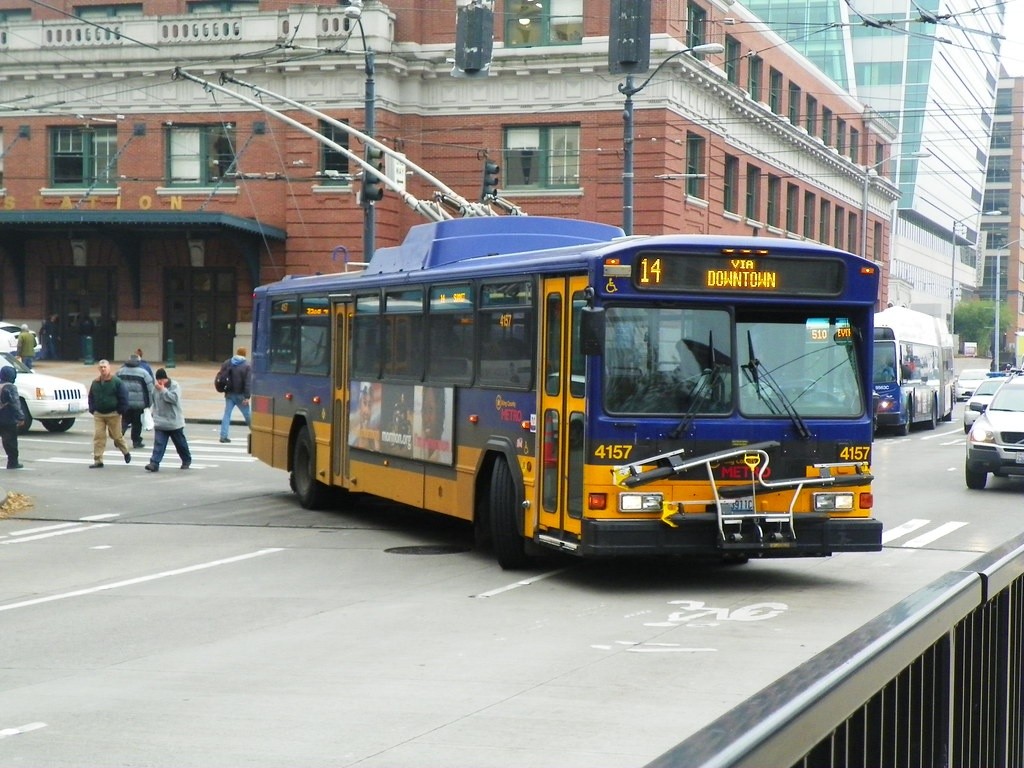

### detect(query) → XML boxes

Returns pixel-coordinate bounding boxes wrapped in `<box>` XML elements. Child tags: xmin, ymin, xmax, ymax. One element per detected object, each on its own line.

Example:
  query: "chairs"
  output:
<box><xmin>611</xmin><ymin>366</ymin><xmax>641</xmax><ymax>411</ymax></box>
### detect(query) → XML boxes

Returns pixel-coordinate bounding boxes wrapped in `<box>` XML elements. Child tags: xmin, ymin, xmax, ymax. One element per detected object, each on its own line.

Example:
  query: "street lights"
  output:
<box><xmin>617</xmin><ymin>43</ymin><xmax>725</xmax><ymax>236</ymax></box>
<box><xmin>951</xmin><ymin>210</ymin><xmax>1002</xmax><ymax>340</ymax></box>
<box><xmin>859</xmin><ymin>152</ymin><xmax>931</xmax><ymax>259</ymax></box>
<box><xmin>342</xmin><ymin>6</ymin><xmax>375</xmax><ymax>263</ymax></box>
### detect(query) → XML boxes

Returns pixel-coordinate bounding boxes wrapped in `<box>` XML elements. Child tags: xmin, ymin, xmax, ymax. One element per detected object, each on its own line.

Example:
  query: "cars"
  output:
<box><xmin>955</xmin><ymin>369</ymin><xmax>992</xmax><ymax>401</ymax></box>
<box><xmin>966</xmin><ymin>369</ymin><xmax>1024</xmax><ymax>490</ymax></box>
<box><xmin>964</xmin><ymin>373</ymin><xmax>1011</xmax><ymax>434</ymax></box>
<box><xmin>0</xmin><ymin>351</ymin><xmax>89</xmax><ymax>432</ymax></box>
<box><xmin>0</xmin><ymin>322</ymin><xmax>42</xmax><ymax>354</ymax></box>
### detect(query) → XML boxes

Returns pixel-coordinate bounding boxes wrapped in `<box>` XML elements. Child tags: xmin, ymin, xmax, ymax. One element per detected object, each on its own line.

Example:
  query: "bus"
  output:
<box><xmin>248</xmin><ymin>215</ymin><xmax>882</xmax><ymax>570</ymax></box>
<box><xmin>802</xmin><ymin>307</ymin><xmax>954</xmax><ymax>437</ymax></box>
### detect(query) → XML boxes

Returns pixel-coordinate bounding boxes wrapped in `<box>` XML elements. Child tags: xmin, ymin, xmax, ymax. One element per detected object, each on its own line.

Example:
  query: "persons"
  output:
<box><xmin>81</xmin><ymin>316</ymin><xmax>94</xmax><ymax>361</ymax></box>
<box><xmin>0</xmin><ymin>366</ymin><xmax>25</xmax><ymax>469</ymax></box>
<box><xmin>219</xmin><ymin>348</ymin><xmax>252</xmax><ymax>443</ymax></box>
<box><xmin>88</xmin><ymin>359</ymin><xmax>131</xmax><ymax>469</ymax></box>
<box><xmin>144</xmin><ymin>368</ymin><xmax>191</xmax><ymax>473</ymax></box>
<box><xmin>135</xmin><ymin>349</ymin><xmax>155</xmax><ymax>386</ymax></box>
<box><xmin>421</xmin><ymin>386</ymin><xmax>445</xmax><ymax>461</ymax></box>
<box><xmin>40</xmin><ymin>314</ymin><xmax>60</xmax><ymax>361</ymax></box>
<box><xmin>17</xmin><ymin>324</ymin><xmax>37</xmax><ymax>370</ymax></box>
<box><xmin>354</xmin><ymin>382</ymin><xmax>375</xmax><ymax>451</ymax></box>
<box><xmin>114</xmin><ymin>354</ymin><xmax>154</xmax><ymax>448</ymax></box>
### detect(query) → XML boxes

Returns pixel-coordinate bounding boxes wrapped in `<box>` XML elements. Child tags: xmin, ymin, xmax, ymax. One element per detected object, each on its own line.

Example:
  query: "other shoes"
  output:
<box><xmin>181</xmin><ymin>457</ymin><xmax>192</xmax><ymax>468</ymax></box>
<box><xmin>144</xmin><ymin>464</ymin><xmax>159</xmax><ymax>471</ymax></box>
<box><xmin>114</xmin><ymin>440</ymin><xmax>119</xmax><ymax>447</ymax></box>
<box><xmin>124</xmin><ymin>453</ymin><xmax>131</xmax><ymax>463</ymax></box>
<box><xmin>134</xmin><ymin>443</ymin><xmax>145</xmax><ymax>448</ymax></box>
<box><xmin>89</xmin><ymin>462</ymin><xmax>104</xmax><ymax>468</ymax></box>
<box><xmin>219</xmin><ymin>438</ymin><xmax>231</xmax><ymax>443</ymax></box>
<box><xmin>7</xmin><ymin>463</ymin><xmax>24</xmax><ymax>469</ymax></box>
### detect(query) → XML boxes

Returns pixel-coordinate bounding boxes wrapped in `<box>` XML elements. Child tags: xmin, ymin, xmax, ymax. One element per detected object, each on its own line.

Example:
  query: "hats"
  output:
<box><xmin>358</xmin><ymin>381</ymin><xmax>375</xmax><ymax>407</ymax></box>
<box><xmin>134</xmin><ymin>348</ymin><xmax>143</xmax><ymax>356</ymax></box>
<box><xmin>155</xmin><ymin>367</ymin><xmax>168</xmax><ymax>379</ymax></box>
<box><xmin>128</xmin><ymin>353</ymin><xmax>141</xmax><ymax>361</ymax></box>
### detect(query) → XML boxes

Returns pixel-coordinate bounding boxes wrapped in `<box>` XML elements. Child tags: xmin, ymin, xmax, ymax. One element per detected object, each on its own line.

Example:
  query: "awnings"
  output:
<box><xmin>0</xmin><ymin>210</ymin><xmax>287</xmax><ymax>244</ymax></box>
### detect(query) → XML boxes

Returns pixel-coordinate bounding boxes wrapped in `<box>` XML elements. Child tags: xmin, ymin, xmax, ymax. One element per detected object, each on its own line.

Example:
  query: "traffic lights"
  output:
<box><xmin>482</xmin><ymin>160</ymin><xmax>499</xmax><ymax>198</ymax></box>
<box><xmin>358</xmin><ymin>144</ymin><xmax>383</xmax><ymax>204</ymax></box>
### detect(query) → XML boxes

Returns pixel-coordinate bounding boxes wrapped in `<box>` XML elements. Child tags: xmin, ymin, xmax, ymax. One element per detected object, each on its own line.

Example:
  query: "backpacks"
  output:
<box><xmin>215</xmin><ymin>359</ymin><xmax>232</xmax><ymax>393</ymax></box>
